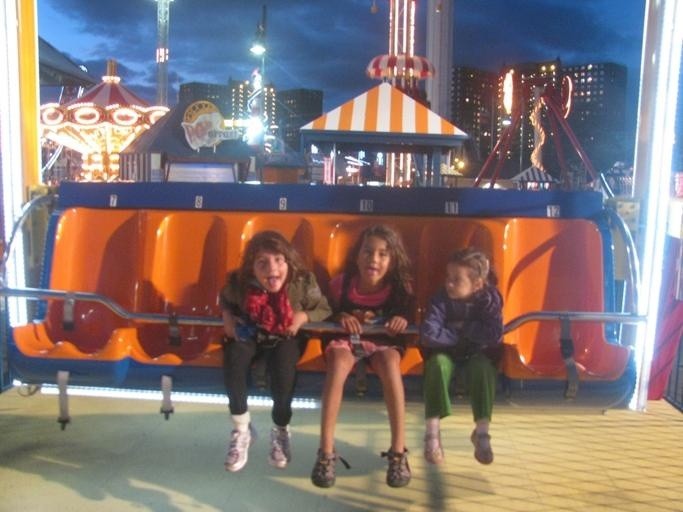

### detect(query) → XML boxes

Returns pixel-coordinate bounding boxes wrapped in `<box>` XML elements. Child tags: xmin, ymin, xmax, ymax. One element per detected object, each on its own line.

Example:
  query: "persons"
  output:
<box><xmin>418</xmin><ymin>246</ymin><xmax>506</xmax><ymax>464</ymax></box>
<box><xmin>310</xmin><ymin>222</ymin><xmax>417</xmax><ymax>488</ymax></box>
<box><xmin>218</xmin><ymin>229</ymin><xmax>333</xmax><ymax>472</ymax></box>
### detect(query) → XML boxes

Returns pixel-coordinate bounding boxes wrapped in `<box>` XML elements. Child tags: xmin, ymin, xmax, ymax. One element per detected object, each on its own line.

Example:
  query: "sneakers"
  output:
<box><xmin>269</xmin><ymin>425</ymin><xmax>290</xmax><ymax>468</ymax></box>
<box><xmin>225</xmin><ymin>424</ymin><xmax>257</xmax><ymax>471</ymax></box>
<box><xmin>312</xmin><ymin>448</ymin><xmax>349</xmax><ymax>488</ymax></box>
<box><xmin>471</xmin><ymin>430</ymin><xmax>493</xmax><ymax>464</ymax></box>
<box><xmin>381</xmin><ymin>447</ymin><xmax>411</xmax><ymax>488</ymax></box>
<box><xmin>424</xmin><ymin>433</ymin><xmax>443</xmax><ymax>463</ymax></box>
